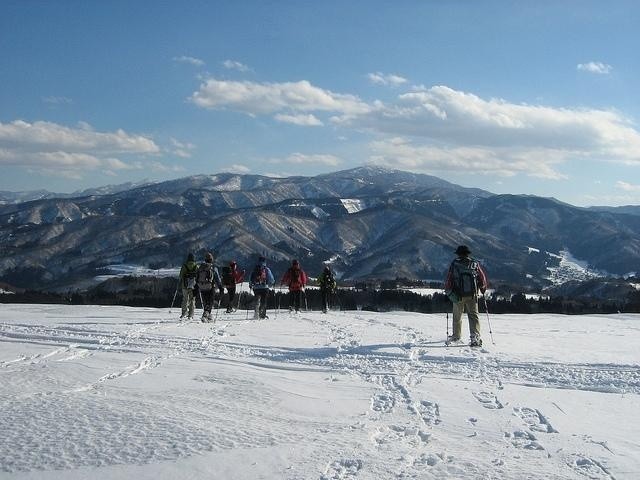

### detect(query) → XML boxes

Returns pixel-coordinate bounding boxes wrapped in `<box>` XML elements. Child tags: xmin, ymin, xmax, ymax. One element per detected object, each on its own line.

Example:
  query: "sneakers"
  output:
<box><xmin>471</xmin><ymin>338</ymin><xmax>483</xmax><ymax>346</ymax></box>
<box><xmin>447</xmin><ymin>335</ymin><xmax>461</xmax><ymax>343</ymax></box>
<box><xmin>178</xmin><ymin>305</ymin><xmax>332</xmax><ymax>323</ymax></box>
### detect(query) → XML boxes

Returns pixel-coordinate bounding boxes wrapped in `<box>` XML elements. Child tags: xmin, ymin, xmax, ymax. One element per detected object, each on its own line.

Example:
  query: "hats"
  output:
<box><xmin>292</xmin><ymin>259</ymin><xmax>300</xmax><ymax>264</ymax></box>
<box><xmin>229</xmin><ymin>259</ymin><xmax>237</xmax><ymax>267</ymax></box>
<box><xmin>204</xmin><ymin>252</ymin><xmax>213</xmax><ymax>261</ymax></box>
<box><xmin>187</xmin><ymin>253</ymin><xmax>195</xmax><ymax>261</ymax></box>
<box><xmin>453</xmin><ymin>245</ymin><xmax>473</xmax><ymax>255</ymax></box>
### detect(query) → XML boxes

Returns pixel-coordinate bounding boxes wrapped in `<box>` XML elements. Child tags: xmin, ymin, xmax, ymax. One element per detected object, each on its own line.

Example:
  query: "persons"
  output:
<box><xmin>316</xmin><ymin>265</ymin><xmax>336</xmax><ymax>314</ymax></box>
<box><xmin>222</xmin><ymin>260</ymin><xmax>245</xmax><ymax>314</ymax></box>
<box><xmin>196</xmin><ymin>252</ymin><xmax>224</xmax><ymax>324</ymax></box>
<box><xmin>282</xmin><ymin>259</ymin><xmax>308</xmax><ymax>313</ymax></box>
<box><xmin>248</xmin><ymin>256</ymin><xmax>276</xmax><ymax>321</ymax></box>
<box><xmin>176</xmin><ymin>252</ymin><xmax>199</xmax><ymax>319</ymax></box>
<box><xmin>444</xmin><ymin>244</ymin><xmax>487</xmax><ymax>345</ymax></box>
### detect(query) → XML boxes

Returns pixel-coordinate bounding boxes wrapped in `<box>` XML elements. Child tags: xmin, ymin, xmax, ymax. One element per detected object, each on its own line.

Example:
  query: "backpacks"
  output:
<box><xmin>254</xmin><ymin>263</ymin><xmax>267</xmax><ymax>285</ymax></box>
<box><xmin>452</xmin><ymin>258</ymin><xmax>479</xmax><ymax>296</ymax></box>
<box><xmin>197</xmin><ymin>262</ymin><xmax>214</xmax><ymax>291</ymax></box>
<box><xmin>323</xmin><ymin>272</ymin><xmax>335</xmax><ymax>289</ymax></box>
<box><xmin>288</xmin><ymin>267</ymin><xmax>302</xmax><ymax>289</ymax></box>
<box><xmin>221</xmin><ymin>266</ymin><xmax>234</xmax><ymax>286</ymax></box>
<box><xmin>183</xmin><ymin>263</ymin><xmax>198</xmax><ymax>290</ymax></box>
